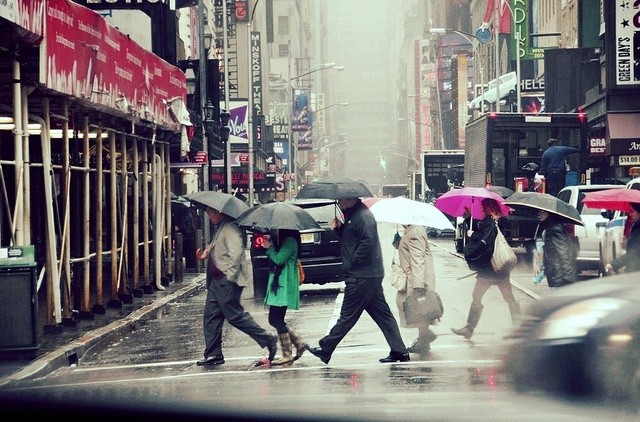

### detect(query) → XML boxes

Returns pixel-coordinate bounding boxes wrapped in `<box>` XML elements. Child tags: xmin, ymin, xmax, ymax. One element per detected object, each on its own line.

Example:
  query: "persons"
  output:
<box><xmin>450</xmin><ymin>198</ymin><xmax>526</xmax><ymax>341</ymax></box>
<box><xmin>538</xmin><ymin>138</ymin><xmax>583</xmax><ymax>197</ymax></box>
<box><xmin>604</xmin><ymin>202</ymin><xmax>639</xmax><ymax>273</ymax></box>
<box><xmin>392</xmin><ymin>218</ymin><xmax>443</xmax><ymax>354</ymax></box>
<box><xmin>527</xmin><ymin>208</ymin><xmax>582</xmax><ymax>289</ymax></box>
<box><xmin>447</xmin><ymin>170</ymin><xmax>461</xmax><ymax>190</ymax></box>
<box><xmin>259</xmin><ymin>222</ymin><xmax>310</xmax><ymax>366</ymax></box>
<box><xmin>197</xmin><ymin>208</ymin><xmax>278</xmax><ymax>370</ymax></box>
<box><xmin>301</xmin><ymin>196</ymin><xmax>411</xmax><ymax>366</ymax></box>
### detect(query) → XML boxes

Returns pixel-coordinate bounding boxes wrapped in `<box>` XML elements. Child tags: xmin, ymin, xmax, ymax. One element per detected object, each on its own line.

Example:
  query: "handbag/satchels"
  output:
<box><xmin>297</xmin><ymin>256</ymin><xmax>305</xmax><ymax>285</ymax></box>
<box><xmin>403</xmin><ymin>289</ymin><xmax>443</xmax><ymax>324</ymax></box>
<box><xmin>490</xmin><ymin>220</ymin><xmax>517</xmax><ymax>275</ymax></box>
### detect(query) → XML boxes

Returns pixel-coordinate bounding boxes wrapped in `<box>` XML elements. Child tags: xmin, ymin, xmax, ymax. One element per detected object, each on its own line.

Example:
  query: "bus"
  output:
<box><xmin>455</xmin><ymin>112</ymin><xmax>587</xmax><ymax>257</ymax></box>
<box><xmin>422</xmin><ymin>153</ymin><xmax>464</xmax><ymax>192</ymax></box>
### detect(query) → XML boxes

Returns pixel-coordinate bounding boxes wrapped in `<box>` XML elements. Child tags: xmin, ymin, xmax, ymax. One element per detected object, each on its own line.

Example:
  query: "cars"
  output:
<box><xmin>506</xmin><ymin>277</ymin><xmax>639</xmax><ymax>412</ymax></box>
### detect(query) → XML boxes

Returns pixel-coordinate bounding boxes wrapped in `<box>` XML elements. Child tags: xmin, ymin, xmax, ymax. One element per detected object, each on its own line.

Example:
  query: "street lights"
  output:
<box><xmin>408</xmin><ymin>93</ymin><xmax>444</xmax><ymax>151</ymax></box>
<box><xmin>289</xmin><ymin>62</ymin><xmax>344</xmax><ymax>193</ymax></box>
<box><xmin>294</xmin><ymin>102</ymin><xmax>348</xmax><ymax>191</ymax></box>
<box><xmin>428</xmin><ymin>27</ymin><xmax>486</xmax><ymax>115</ymax></box>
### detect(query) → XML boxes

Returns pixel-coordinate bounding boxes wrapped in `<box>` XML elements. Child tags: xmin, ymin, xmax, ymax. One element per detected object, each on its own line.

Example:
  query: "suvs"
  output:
<box><xmin>599</xmin><ymin>178</ymin><xmax>640</xmax><ymax>271</ymax></box>
<box><xmin>535</xmin><ymin>184</ymin><xmax>627</xmax><ymax>273</ymax></box>
<box><xmin>249</xmin><ymin>200</ymin><xmax>355</xmax><ymax>296</ymax></box>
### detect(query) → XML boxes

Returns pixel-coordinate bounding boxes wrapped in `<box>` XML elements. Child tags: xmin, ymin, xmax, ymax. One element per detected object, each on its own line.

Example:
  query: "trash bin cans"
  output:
<box><xmin>0</xmin><ymin>245</ymin><xmax>43</xmax><ymax>361</ymax></box>
<box><xmin>182</xmin><ymin>238</ymin><xmax>204</xmax><ymax>268</ymax></box>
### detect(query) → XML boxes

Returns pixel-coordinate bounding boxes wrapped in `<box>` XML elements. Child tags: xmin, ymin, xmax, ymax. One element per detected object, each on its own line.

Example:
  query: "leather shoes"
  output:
<box><xmin>197</xmin><ymin>354</ymin><xmax>225</xmax><ymax>365</ymax></box>
<box><xmin>308</xmin><ymin>347</ymin><xmax>331</xmax><ymax>364</ymax></box>
<box><xmin>380</xmin><ymin>352</ymin><xmax>409</xmax><ymax>362</ymax></box>
<box><xmin>267</xmin><ymin>333</ymin><xmax>277</xmax><ymax>361</ymax></box>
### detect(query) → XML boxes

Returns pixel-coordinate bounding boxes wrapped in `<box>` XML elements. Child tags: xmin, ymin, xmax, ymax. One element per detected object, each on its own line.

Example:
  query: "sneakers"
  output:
<box><xmin>407</xmin><ymin>338</ymin><xmax>431</xmax><ymax>353</ymax></box>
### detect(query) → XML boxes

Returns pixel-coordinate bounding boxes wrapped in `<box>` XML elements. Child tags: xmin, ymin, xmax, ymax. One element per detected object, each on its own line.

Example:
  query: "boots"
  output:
<box><xmin>285</xmin><ymin>324</ymin><xmax>309</xmax><ymax>361</ymax></box>
<box><xmin>271</xmin><ymin>332</ymin><xmax>293</xmax><ymax>366</ymax></box>
<box><xmin>503</xmin><ymin>302</ymin><xmax>523</xmax><ymax>339</ymax></box>
<box><xmin>451</xmin><ymin>304</ymin><xmax>483</xmax><ymax>340</ymax></box>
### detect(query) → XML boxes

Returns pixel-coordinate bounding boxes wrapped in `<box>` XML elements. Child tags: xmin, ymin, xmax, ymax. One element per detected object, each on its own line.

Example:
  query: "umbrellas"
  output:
<box><xmin>502</xmin><ymin>190</ymin><xmax>585</xmax><ymax>239</ymax></box>
<box><xmin>293</xmin><ymin>175</ymin><xmax>373</xmax><ymax>217</ymax></box>
<box><xmin>368</xmin><ymin>194</ymin><xmax>456</xmax><ymax>264</ymax></box>
<box><xmin>241</xmin><ymin>201</ymin><xmax>319</xmax><ymax>231</ymax></box>
<box><xmin>582</xmin><ymin>187</ymin><xmax>640</xmax><ymax>211</ymax></box>
<box><xmin>432</xmin><ymin>187</ymin><xmax>513</xmax><ymax>236</ymax></box>
<box><xmin>181</xmin><ymin>188</ymin><xmax>254</xmax><ymax>260</ymax></box>
